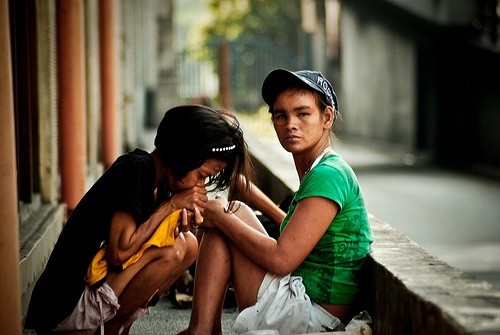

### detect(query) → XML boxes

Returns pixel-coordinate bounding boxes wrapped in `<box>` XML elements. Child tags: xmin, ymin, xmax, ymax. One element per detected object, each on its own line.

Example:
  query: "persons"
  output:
<box><xmin>23</xmin><ymin>105</ymin><xmax>249</xmax><ymax>335</ymax></box>
<box><xmin>177</xmin><ymin>68</ymin><xmax>375</xmax><ymax>335</ymax></box>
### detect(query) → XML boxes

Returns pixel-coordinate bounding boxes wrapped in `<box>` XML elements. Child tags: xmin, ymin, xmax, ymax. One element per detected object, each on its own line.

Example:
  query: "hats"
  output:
<box><xmin>262</xmin><ymin>68</ymin><xmax>338</xmax><ymax>126</ymax></box>
